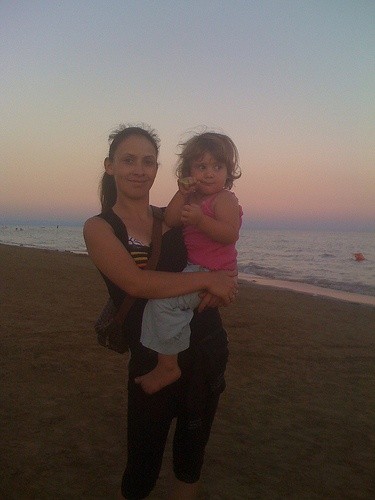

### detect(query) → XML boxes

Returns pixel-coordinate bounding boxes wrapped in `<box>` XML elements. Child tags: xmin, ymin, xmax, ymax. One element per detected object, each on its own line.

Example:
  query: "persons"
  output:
<box><xmin>84</xmin><ymin>124</ymin><xmax>240</xmax><ymax>500</ymax></box>
<box><xmin>135</xmin><ymin>134</ymin><xmax>244</xmax><ymax>395</ymax></box>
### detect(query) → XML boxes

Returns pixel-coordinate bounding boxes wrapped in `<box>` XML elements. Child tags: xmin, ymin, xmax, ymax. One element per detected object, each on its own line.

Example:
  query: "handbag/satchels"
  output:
<box><xmin>94</xmin><ymin>297</ymin><xmax>128</xmax><ymax>354</ymax></box>
